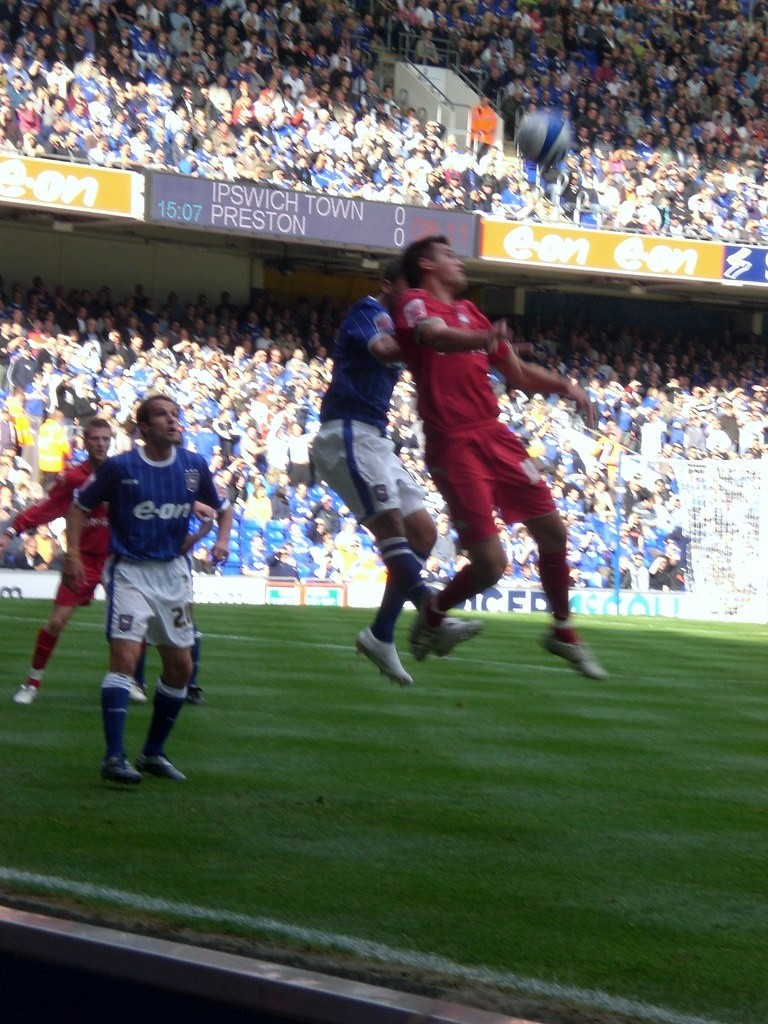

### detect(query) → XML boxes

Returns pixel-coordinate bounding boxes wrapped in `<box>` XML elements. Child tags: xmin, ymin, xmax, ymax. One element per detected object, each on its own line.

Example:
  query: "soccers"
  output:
<box><xmin>518</xmin><ymin>108</ymin><xmax>570</xmax><ymax>164</ymax></box>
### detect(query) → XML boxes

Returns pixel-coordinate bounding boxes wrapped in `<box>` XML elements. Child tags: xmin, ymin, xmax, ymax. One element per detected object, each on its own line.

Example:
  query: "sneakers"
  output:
<box><xmin>128</xmin><ymin>678</ymin><xmax>148</xmax><ymax>703</ymax></box>
<box><xmin>135</xmin><ymin>752</ymin><xmax>188</xmax><ymax>781</ymax></box>
<box><xmin>186</xmin><ymin>683</ymin><xmax>205</xmax><ymax>704</ymax></box>
<box><xmin>433</xmin><ymin>617</ymin><xmax>485</xmax><ymax>658</ymax></box>
<box><xmin>355</xmin><ymin>625</ymin><xmax>413</xmax><ymax>686</ymax></box>
<box><xmin>412</xmin><ymin>590</ymin><xmax>445</xmax><ymax>661</ymax></box>
<box><xmin>137</xmin><ymin>682</ymin><xmax>148</xmax><ymax>695</ymax></box>
<box><xmin>99</xmin><ymin>751</ymin><xmax>144</xmax><ymax>784</ymax></box>
<box><xmin>536</xmin><ymin>630</ymin><xmax>609</xmax><ymax>680</ymax></box>
<box><xmin>13</xmin><ymin>684</ymin><xmax>38</xmax><ymax>704</ymax></box>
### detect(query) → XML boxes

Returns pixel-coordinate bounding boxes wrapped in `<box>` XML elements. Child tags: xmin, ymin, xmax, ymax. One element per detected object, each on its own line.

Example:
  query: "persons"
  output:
<box><xmin>0</xmin><ymin>0</ymin><xmax>768</xmax><ymax>246</ymax></box>
<box><xmin>394</xmin><ymin>234</ymin><xmax>610</xmax><ymax>681</ymax></box>
<box><xmin>0</xmin><ymin>417</ymin><xmax>148</xmax><ymax>704</ymax></box>
<box><xmin>314</xmin><ymin>259</ymin><xmax>485</xmax><ymax>685</ymax></box>
<box><xmin>1</xmin><ymin>272</ymin><xmax>768</xmax><ymax>599</ymax></box>
<box><xmin>70</xmin><ymin>394</ymin><xmax>233</xmax><ymax>784</ymax></box>
